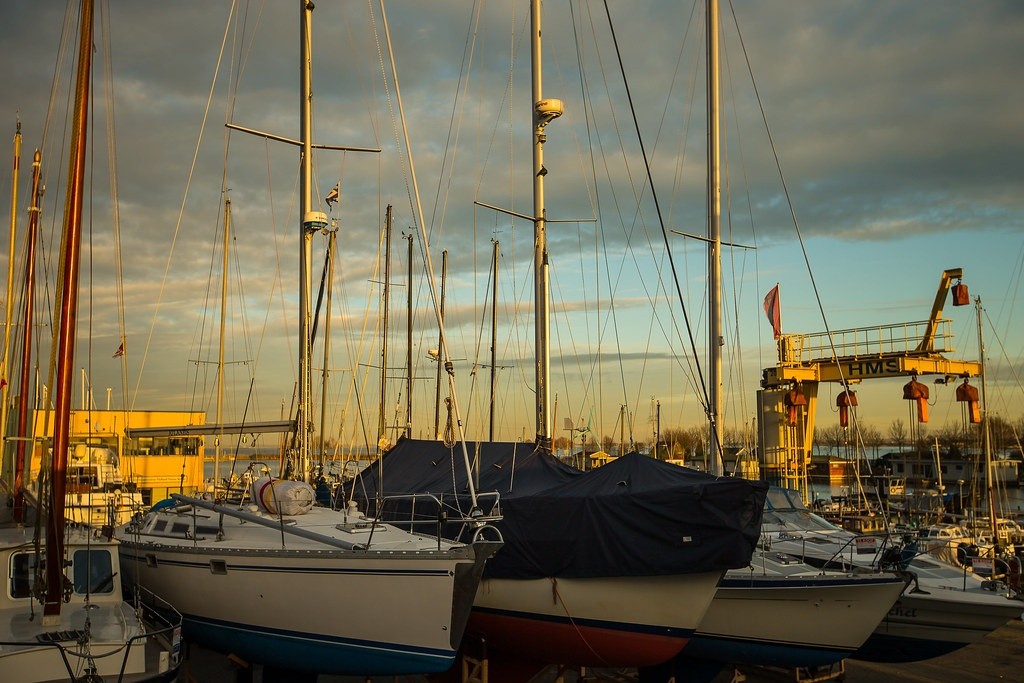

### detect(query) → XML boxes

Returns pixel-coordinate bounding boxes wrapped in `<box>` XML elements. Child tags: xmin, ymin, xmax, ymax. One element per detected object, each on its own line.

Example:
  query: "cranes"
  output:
<box><xmin>755</xmin><ymin>268</ymin><xmax>983</xmax><ymax>507</ymax></box>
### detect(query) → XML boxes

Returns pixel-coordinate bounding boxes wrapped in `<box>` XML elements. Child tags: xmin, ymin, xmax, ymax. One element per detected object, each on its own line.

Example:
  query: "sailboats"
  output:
<box><xmin>0</xmin><ymin>0</ymin><xmax>1024</xmax><ymax>683</ymax></box>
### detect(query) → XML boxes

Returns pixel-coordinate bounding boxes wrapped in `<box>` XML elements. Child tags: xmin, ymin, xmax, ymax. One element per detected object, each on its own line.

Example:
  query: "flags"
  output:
<box><xmin>763</xmin><ymin>285</ymin><xmax>782</xmax><ymax>340</ymax></box>
<box><xmin>112</xmin><ymin>342</ymin><xmax>124</xmax><ymax>359</ymax></box>
